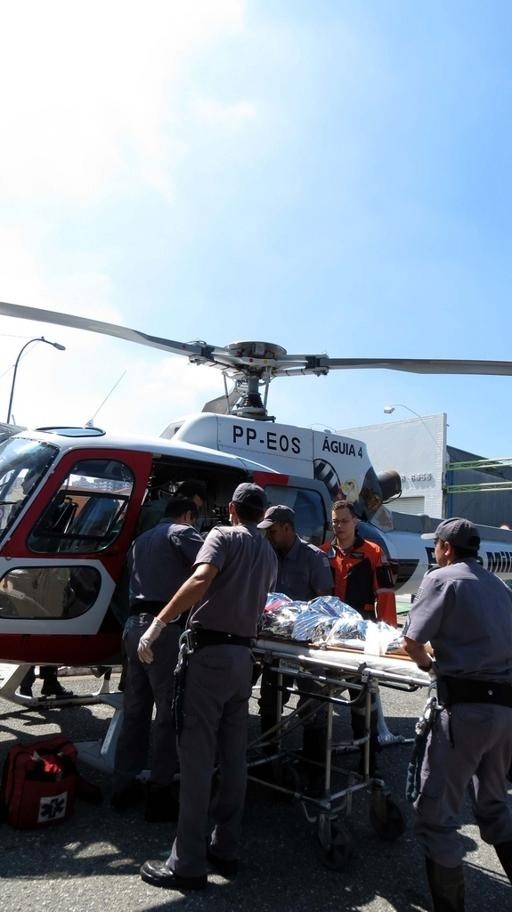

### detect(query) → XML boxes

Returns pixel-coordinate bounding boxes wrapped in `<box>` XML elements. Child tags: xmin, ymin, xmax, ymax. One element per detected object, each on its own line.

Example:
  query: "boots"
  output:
<box><xmin>492</xmin><ymin>839</ymin><xmax>512</xmax><ymax>886</ymax></box>
<box><xmin>423</xmin><ymin>852</ymin><xmax>465</xmax><ymax>911</ymax></box>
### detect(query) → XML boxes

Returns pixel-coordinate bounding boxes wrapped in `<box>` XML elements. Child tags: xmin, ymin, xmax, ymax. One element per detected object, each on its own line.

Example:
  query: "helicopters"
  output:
<box><xmin>1</xmin><ymin>301</ymin><xmax>510</xmax><ymax>776</ymax></box>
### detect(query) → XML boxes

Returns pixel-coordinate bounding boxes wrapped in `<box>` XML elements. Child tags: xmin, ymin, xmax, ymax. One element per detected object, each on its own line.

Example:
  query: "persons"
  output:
<box><xmin>256</xmin><ymin>504</ymin><xmax>336</xmax><ymax>762</ymax></box>
<box><xmin>18</xmin><ymin>665</ymin><xmax>73</xmax><ymax>699</ymax></box>
<box><xmin>134</xmin><ymin>482</ymin><xmax>279</xmax><ymax>891</ymax></box>
<box><xmin>104</xmin><ymin>498</ymin><xmax>204</xmax><ymax>810</ymax></box>
<box><xmin>396</xmin><ymin>517</ymin><xmax>512</xmax><ymax>912</ymax></box>
<box><xmin>9</xmin><ymin>469</ymin><xmax>63</xmax><ymax>552</ymax></box>
<box><xmin>137</xmin><ymin>480</ymin><xmax>205</xmax><ymax>534</ymax></box>
<box><xmin>317</xmin><ymin>500</ymin><xmax>397</xmax><ymax>780</ymax></box>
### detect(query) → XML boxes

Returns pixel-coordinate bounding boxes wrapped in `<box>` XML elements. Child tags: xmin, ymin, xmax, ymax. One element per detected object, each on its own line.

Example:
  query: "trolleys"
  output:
<box><xmin>239</xmin><ymin>628</ymin><xmax>436</xmax><ymax>864</ymax></box>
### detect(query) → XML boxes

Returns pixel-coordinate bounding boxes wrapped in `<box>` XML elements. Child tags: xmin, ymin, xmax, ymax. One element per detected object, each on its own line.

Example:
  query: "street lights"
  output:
<box><xmin>6</xmin><ymin>335</ymin><xmax>66</xmax><ymax>426</ymax></box>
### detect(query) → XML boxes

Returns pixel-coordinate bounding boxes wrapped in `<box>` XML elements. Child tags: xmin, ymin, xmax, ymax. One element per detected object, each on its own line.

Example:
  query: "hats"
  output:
<box><xmin>419</xmin><ymin>516</ymin><xmax>480</xmax><ymax>551</ymax></box>
<box><xmin>255</xmin><ymin>504</ymin><xmax>296</xmax><ymax>530</ymax></box>
<box><xmin>230</xmin><ymin>482</ymin><xmax>267</xmax><ymax>511</ymax></box>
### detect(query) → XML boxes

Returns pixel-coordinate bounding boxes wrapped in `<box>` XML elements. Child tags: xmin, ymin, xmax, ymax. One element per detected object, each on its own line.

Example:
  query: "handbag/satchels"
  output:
<box><xmin>0</xmin><ymin>732</ymin><xmax>85</xmax><ymax>829</ymax></box>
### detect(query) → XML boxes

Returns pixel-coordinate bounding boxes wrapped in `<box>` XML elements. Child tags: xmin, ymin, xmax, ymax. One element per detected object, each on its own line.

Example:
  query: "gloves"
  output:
<box><xmin>135</xmin><ymin>615</ymin><xmax>168</xmax><ymax>666</ymax></box>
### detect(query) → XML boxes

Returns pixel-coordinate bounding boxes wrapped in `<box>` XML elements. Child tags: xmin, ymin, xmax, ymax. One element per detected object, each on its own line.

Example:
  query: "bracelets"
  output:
<box><xmin>418</xmin><ymin>662</ymin><xmax>433</xmax><ymax>673</ymax></box>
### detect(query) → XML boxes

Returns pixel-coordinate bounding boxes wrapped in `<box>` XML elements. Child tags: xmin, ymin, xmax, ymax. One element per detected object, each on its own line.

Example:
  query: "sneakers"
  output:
<box><xmin>205</xmin><ymin>843</ymin><xmax>241</xmax><ymax>881</ymax></box>
<box><xmin>139</xmin><ymin>856</ymin><xmax>208</xmax><ymax>890</ymax></box>
<box><xmin>143</xmin><ymin>788</ymin><xmax>181</xmax><ymax>823</ymax></box>
<box><xmin>20</xmin><ymin>684</ymin><xmax>32</xmax><ymax>696</ymax></box>
<box><xmin>41</xmin><ymin>680</ymin><xmax>74</xmax><ymax>697</ymax></box>
<box><xmin>110</xmin><ymin>790</ymin><xmax>144</xmax><ymax>815</ymax></box>
